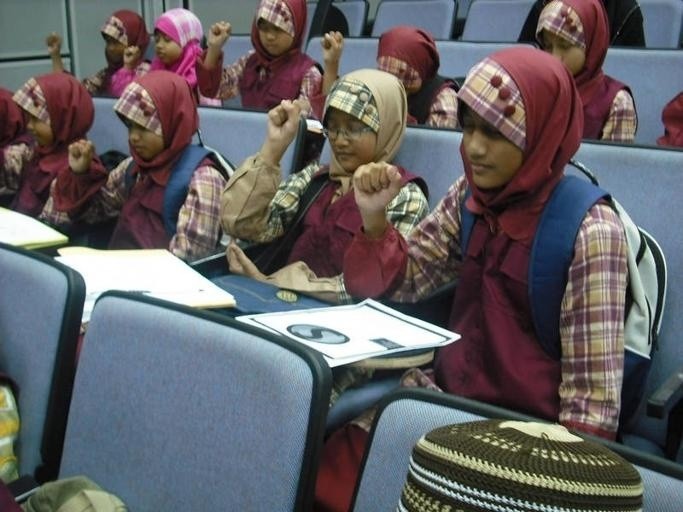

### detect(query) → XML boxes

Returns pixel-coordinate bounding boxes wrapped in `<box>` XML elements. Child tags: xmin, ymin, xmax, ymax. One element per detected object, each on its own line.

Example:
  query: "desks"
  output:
<box><xmin>208</xmin><ymin>273</ymin><xmax>403</xmax><ymax>430</ymax></box>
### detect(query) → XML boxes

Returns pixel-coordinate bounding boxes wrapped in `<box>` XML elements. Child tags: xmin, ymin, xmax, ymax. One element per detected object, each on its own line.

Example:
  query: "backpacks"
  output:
<box><xmin>459</xmin><ymin>159</ymin><xmax>668</xmax><ymax>423</ymax></box>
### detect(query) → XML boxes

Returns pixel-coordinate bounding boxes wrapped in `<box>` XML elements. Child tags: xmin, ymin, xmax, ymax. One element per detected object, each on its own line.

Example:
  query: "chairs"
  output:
<box><xmin>0</xmin><ymin>242</ymin><xmax>87</xmax><ymax>512</ymax></box>
<box><xmin>561</xmin><ymin>142</ymin><xmax>682</xmax><ymax>463</ymax></box>
<box><xmin>347</xmin><ymin>390</ymin><xmax>682</xmax><ymax>512</ymax></box>
<box><xmin>2</xmin><ymin>2</ymin><xmax>683</xmax><ymax>273</ymax></box>
<box><xmin>1</xmin><ymin>290</ymin><xmax>333</xmax><ymax>512</ymax></box>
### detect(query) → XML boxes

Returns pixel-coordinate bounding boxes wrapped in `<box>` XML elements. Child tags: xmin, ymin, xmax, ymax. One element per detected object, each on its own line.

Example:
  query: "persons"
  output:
<box><xmin>1</xmin><ymin>0</ymin><xmax>683</xmax><ymax>510</ymax></box>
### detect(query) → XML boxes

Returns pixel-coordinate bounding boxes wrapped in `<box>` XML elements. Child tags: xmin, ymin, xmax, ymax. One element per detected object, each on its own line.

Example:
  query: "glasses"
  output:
<box><xmin>320</xmin><ymin>127</ymin><xmax>371</xmax><ymax>140</ymax></box>
